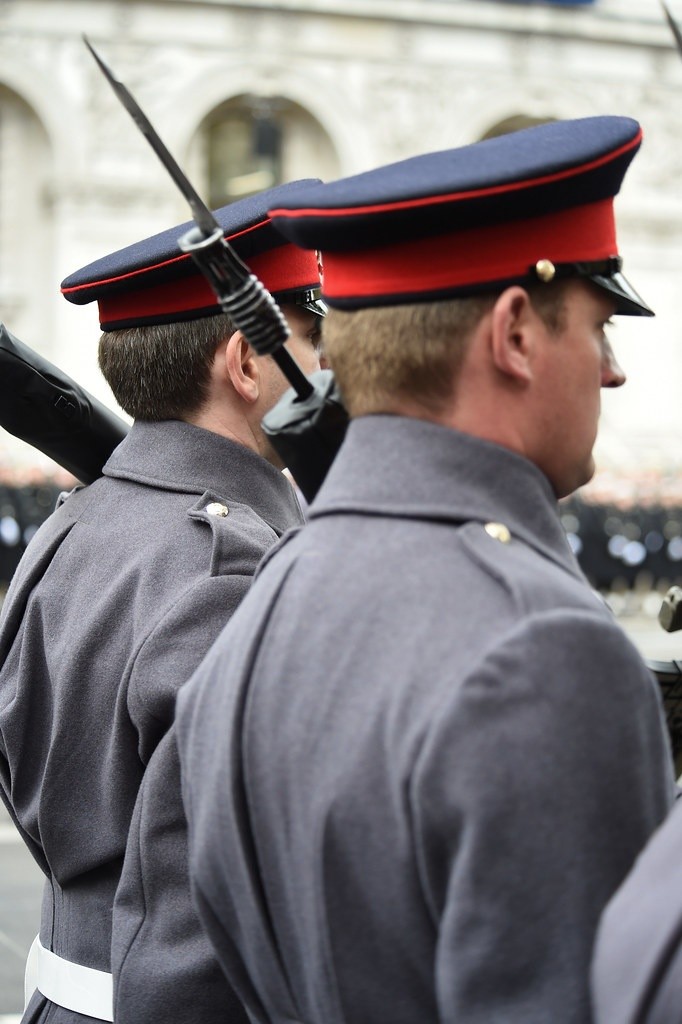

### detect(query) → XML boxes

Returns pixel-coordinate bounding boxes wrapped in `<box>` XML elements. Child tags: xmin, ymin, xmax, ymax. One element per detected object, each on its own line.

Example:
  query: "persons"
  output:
<box><xmin>110</xmin><ymin>113</ymin><xmax>677</xmax><ymax>1024</ymax></box>
<box><xmin>0</xmin><ymin>179</ymin><xmax>333</xmax><ymax>1024</ymax></box>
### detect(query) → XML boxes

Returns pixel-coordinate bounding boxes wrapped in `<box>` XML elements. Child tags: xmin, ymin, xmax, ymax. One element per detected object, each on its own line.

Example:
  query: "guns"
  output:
<box><xmin>0</xmin><ymin>331</ymin><xmax>133</xmax><ymax>488</ymax></box>
<box><xmin>78</xmin><ymin>23</ymin><xmax>682</xmax><ymax>783</ymax></box>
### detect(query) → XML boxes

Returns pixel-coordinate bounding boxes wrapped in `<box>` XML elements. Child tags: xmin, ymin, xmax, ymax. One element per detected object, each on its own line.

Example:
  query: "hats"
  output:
<box><xmin>60</xmin><ymin>177</ymin><xmax>327</xmax><ymax>332</ymax></box>
<box><xmin>268</xmin><ymin>117</ymin><xmax>657</xmax><ymax>318</ymax></box>
